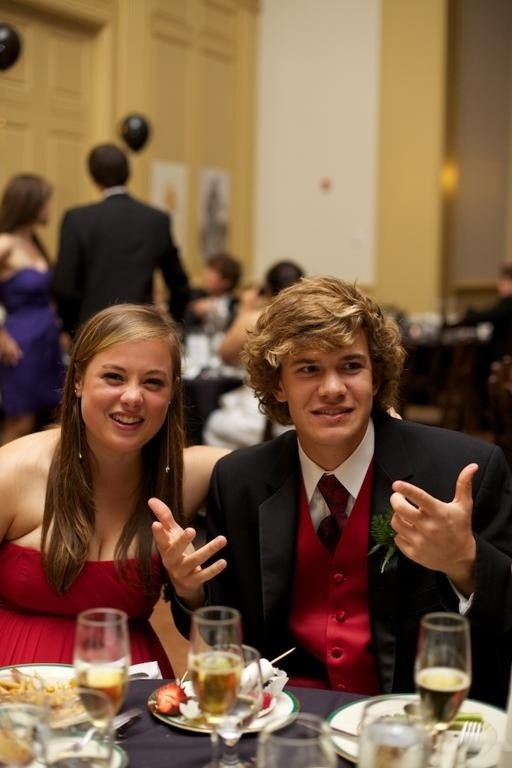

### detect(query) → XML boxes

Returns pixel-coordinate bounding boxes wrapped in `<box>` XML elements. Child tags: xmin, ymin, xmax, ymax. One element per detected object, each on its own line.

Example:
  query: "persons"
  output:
<box><xmin>182</xmin><ymin>255</ymin><xmax>242</xmax><ymax>337</ymax></box>
<box><xmin>0</xmin><ymin>304</ymin><xmax>403</xmax><ymax>681</ymax></box>
<box><xmin>0</xmin><ymin>174</ymin><xmax>72</xmax><ymax>446</ymax></box>
<box><xmin>202</xmin><ymin>262</ymin><xmax>304</xmax><ymax>453</ymax></box>
<box><xmin>147</xmin><ymin>275</ymin><xmax>512</xmax><ymax>712</ymax></box>
<box><xmin>438</xmin><ymin>261</ymin><xmax>512</xmax><ymax>434</ymax></box>
<box><xmin>50</xmin><ymin>144</ymin><xmax>192</xmax><ymax>348</ymax></box>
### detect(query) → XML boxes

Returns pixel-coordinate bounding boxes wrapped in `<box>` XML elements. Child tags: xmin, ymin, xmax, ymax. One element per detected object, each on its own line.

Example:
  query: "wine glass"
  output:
<box><xmin>187</xmin><ymin>604</ymin><xmax>244</xmax><ymax>766</ymax></box>
<box><xmin>189</xmin><ymin>641</ymin><xmax>265</xmax><ymax>768</ymax></box>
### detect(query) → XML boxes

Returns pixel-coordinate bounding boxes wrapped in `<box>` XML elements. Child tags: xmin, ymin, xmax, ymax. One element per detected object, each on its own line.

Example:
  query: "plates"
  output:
<box><xmin>0</xmin><ymin>661</ymin><xmax>74</xmax><ymax>691</ymax></box>
<box><xmin>27</xmin><ymin>732</ymin><xmax>129</xmax><ymax>767</ymax></box>
<box><xmin>325</xmin><ymin>696</ymin><xmax>508</xmax><ymax>764</ymax></box>
<box><xmin>145</xmin><ymin>681</ymin><xmax>300</xmax><ymax>736</ymax></box>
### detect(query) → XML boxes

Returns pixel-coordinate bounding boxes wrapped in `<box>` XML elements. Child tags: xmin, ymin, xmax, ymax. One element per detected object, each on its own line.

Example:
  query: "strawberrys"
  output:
<box><xmin>251</xmin><ymin>690</ymin><xmax>271</xmax><ymax>709</ymax></box>
<box><xmin>155</xmin><ymin>683</ymin><xmax>187</xmax><ymax>716</ymax></box>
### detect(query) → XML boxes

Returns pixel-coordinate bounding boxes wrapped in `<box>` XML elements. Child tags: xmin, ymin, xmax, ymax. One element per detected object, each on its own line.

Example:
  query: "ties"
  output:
<box><xmin>314</xmin><ymin>475</ymin><xmax>350</xmax><ymax>557</ymax></box>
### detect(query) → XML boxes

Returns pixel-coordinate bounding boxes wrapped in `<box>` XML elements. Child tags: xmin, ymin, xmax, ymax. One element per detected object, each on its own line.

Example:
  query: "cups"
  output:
<box><xmin>38</xmin><ymin>690</ymin><xmax>117</xmax><ymax>768</ymax></box>
<box><xmin>255</xmin><ymin>711</ymin><xmax>337</xmax><ymax>768</ymax></box>
<box><xmin>356</xmin><ymin>694</ymin><xmax>433</xmax><ymax>768</ymax></box>
<box><xmin>411</xmin><ymin>612</ymin><xmax>472</xmax><ymax>723</ymax></box>
<box><xmin>72</xmin><ymin>606</ymin><xmax>126</xmax><ymax>727</ymax></box>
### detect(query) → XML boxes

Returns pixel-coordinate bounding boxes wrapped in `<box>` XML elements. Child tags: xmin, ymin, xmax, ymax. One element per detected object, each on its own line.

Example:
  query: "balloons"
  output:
<box><xmin>0</xmin><ymin>22</ymin><xmax>22</xmax><ymax>70</ymax></box>
<box><xmin>120</xmin><ymin>115</ymin><xmax>147</xmax><ymax>153</ymax></box>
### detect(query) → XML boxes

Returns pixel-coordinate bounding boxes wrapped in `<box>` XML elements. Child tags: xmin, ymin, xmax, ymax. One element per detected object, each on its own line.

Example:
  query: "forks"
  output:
<box><xmin>452</xmin><ymin>718</ymin><xmax>490</xmax><ymax>768</ymax></box>
